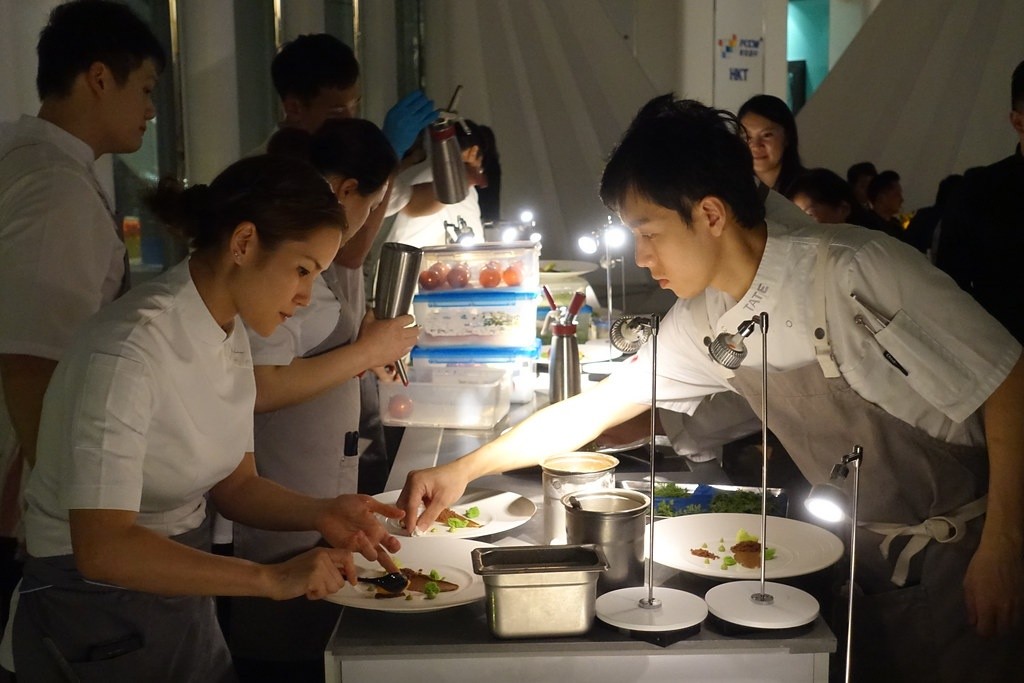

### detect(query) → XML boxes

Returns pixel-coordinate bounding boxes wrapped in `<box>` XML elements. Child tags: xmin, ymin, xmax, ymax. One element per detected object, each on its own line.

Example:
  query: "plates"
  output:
<box><xmin>501</xmin><ymin>427</ymin><xmax>651</xmax><ymax>455</ymax></box>
<box><xmin>645</xmin><ymin>512</ymin><xmax>845</xmax><ymax>580</ymax></box>
<box><xmin>369</xmin><ymin>486</ymin><xmax>538</xmax><ymax>540</ymax></box>
<box><xmin>317</xmin><ymin>536</ymin><xmax>499</xmax><ymax>614</ymax></box>
<box><xmin>536</xmin><ymin>346</ymin><xmax>622</xmax><ymax>365</ymax></box>
<box><xmin>583</xmin><ymin>362</ymin><xmax>622</xmax><ymax>374</ymax></box>
<box><xmin>538</xmin><ymin>259</ymin><xmax>600</xmax><ymax>280</ymax></box>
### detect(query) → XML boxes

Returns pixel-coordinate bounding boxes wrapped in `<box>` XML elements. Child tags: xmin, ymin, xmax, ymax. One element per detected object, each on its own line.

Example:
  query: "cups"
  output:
<box><xmin>538</xmin><ymin>451</ymin><xmax>620</xmax><ymax>545</ymax></box>
<box><xmin>373</xmin><ymin>241</ymin><xmax>424</xmax><ymax>319</ymax></box>
<box><xmin>561</xmin><ymin>486</ymin><xmax>652</xmax><ymax>592</ymax></box>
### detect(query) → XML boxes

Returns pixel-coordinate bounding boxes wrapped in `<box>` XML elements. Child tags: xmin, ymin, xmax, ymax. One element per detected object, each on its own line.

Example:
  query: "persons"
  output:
<box><xmin>0</xmin><ymin>153</ymin><xmax>407</xmax><ymax>683</ymax></box>
<box><xmin>256</xmin><ymin>32</ymin><xmax>483</xmax><ymax>463</ymax></box>
<box><xmin>788</xmin><ymin>168</ymin><xmax>855</xmax><ymax>225</ymax></box>
<box><xmin>844</xmin><ymin>161</ymin><xmax>879</xmax><ymax>225</ymax></box>
<box><xmin>213</xmin><ymin>118</ymin><xmax>420</xmax><ymax>683</ymax></box>
<box><xmin>389</xmin><ymin>118</ymin><xmax>487</xmax><ymax>250</ymax></box>
<box><xmin>901</xmin><ymin>175</ymin><xmax>964</xmax><ymax>252</ymax></box>
<box><xmin>734</xmin><ymin>95</ymin><xmax>811</xmax><ymax>196</ymax></box>
<box><xmin>396</xmin><ymin>92</ymin><xmax>1024</xmax><ymax>683</ymax></box>
<box><xmin>0</xmin><ymin>0</ymin><xmax>169</xmax><ymax>538</ymax></box>
<box><xmin>859</xmin><ymin>170</ymin><xmax>904</xmax><ymax>238</ymax></box>
<box><xmin>932</xmin><ymin>60</ymin><xmax>1024</xmax><ymax>349</ymax></box>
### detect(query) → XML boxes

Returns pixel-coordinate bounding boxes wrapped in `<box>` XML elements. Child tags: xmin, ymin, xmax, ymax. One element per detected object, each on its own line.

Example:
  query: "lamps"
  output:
<box><xmin>705</xmin><ymin>311</ymin><xmax>821</xmax><ymax>629</ymax></box>
<box><xmin>443</xmin><ymin>217</ymin><xmax>474</xmax><ymax>244</ymax></box>
<box><xmin>804</xmin><ymin>444</ymin><xmax>862</xmax><ymax>683</ymax></box>
<box><xmin>578</xmin><ymin>215</ymin><xmax>626</xmax><ymax>375</ymax></box>
<box><xmin>594</xmin><ymin>312</ymin><xmax>709</xmax><ymax>633</ymax></box>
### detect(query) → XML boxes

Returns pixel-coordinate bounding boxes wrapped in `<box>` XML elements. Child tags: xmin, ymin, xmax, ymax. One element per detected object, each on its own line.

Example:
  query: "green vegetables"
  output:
<box><xmin>646</xmin><ymin>481</ymin><xmax>785</xmax><ymax>516</ymax></box>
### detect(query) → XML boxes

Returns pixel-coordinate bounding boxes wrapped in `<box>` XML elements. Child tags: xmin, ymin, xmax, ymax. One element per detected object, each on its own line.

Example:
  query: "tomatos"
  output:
<box><xmin>419</xmin><ymin>260</ymin><xmax>524</xmax><ymax>289</ymax></box>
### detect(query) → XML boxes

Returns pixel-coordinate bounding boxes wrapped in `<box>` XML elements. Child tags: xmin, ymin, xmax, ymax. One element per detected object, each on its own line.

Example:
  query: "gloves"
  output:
<box><xmin>381</xmin><ymin>88</ymin><xmax>440</xmax><ymax>160</ymax></box>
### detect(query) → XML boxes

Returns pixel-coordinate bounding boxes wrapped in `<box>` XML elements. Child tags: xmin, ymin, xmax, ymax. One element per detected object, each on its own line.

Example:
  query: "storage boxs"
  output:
<box><xmin>375</xmin><ymin>238</ymin><xmax>537</xmax><ymax>430</ymax></box>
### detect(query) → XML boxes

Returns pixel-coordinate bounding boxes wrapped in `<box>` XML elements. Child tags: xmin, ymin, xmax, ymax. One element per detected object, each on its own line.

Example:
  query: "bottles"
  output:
<box><xmin>541</xmin><ymin>284</ymin><xmax>587</xmax><ymax>405</ymax></box>
<box><xmin>428</xmin><ymin>119</ymin><xmax>470</xmax><ymax>204</ymax></box>
